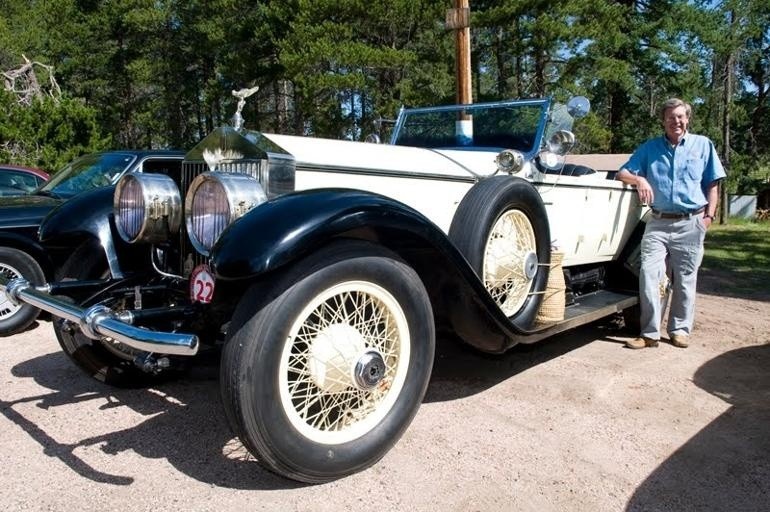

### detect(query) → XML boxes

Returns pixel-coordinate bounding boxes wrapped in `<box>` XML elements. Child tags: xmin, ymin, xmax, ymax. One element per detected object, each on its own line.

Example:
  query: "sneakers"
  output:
<box><xmin>625</xmin><ymin>332</ymin><xmax>659</xmax><ymax>348</ymax></box>
<box><xmin>670</xmin><ymin>335</ymin><xmax>690</xmax><ymax>349</ymax></box>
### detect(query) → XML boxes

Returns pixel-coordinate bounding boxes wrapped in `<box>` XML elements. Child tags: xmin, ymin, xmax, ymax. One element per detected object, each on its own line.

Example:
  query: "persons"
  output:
<box><xmin>616</xmin><ymin>99</ymin><xmax>727</xmax><ymax>350</ymax></box>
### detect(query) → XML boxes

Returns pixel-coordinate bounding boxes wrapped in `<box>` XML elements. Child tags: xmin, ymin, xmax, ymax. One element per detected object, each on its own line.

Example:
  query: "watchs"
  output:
<box><xmin>704</xmin><ymin>215</ymin><xmax>717</xmax><ymax>223</ymax></box>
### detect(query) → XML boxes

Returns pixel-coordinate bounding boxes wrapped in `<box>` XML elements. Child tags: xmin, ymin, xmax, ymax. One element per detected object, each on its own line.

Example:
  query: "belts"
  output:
<box><xmin>651</xmin><ymin>206</ymin><xmax>707</xmax><ymax>221</ymax></box>
